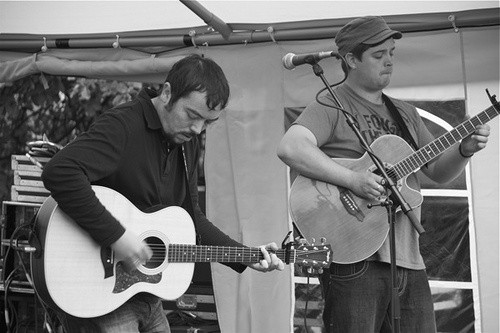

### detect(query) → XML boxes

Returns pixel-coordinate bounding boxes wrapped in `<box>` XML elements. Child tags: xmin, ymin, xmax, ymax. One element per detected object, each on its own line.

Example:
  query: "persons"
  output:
<box><xmin>276</xmin><ymin>17</ymin><xmax>491</xmax><ymax>333</ymax></box>
<box><xmin>38</xmin><ymin>56</ymin><xmax>284</xmax><ymax>333</ymax></box>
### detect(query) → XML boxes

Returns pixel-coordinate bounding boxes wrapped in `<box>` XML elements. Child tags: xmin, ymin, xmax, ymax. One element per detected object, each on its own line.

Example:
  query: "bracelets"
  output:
<box><xmin>458</xmin><ymin>143</ymin><xmax>474</xmax><ymax>158</ymax></box>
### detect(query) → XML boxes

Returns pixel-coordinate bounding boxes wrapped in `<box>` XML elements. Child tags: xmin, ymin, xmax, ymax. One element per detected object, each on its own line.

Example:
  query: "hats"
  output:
<box><xmin>335</xmin><ymin>16</ymin><xmax>402</xmax><ymax>57</ymax></box>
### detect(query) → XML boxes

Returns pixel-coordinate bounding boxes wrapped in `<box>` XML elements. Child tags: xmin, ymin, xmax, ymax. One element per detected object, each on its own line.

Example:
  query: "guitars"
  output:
<box><xmin>287</xmin><ymin>87</ymin><xmax>500</xmax><ymax>265</ymax></box>
<box><xmin>29</xmin><ymin>185</ymin><xmax>334</xmax><ymax>320</ymax></box>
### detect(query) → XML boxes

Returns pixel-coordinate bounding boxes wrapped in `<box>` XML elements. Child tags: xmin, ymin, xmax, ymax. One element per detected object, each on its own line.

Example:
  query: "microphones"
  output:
<box><xmin>282</xmin><ymin>51</ymin><xmax>337</xmax><ymax>70</ymax></box>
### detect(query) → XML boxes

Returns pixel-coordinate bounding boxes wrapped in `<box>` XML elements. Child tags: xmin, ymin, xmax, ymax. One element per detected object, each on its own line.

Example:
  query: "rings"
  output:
<box><xmin>377</xmin><ymin>185</ymin><xmax>381</xmax><ymax>191</ymax></box>
<box><xmin>132</xmin><ymin>258</ymin><xmax>138</xmax><ymax>264</ymax></box>
